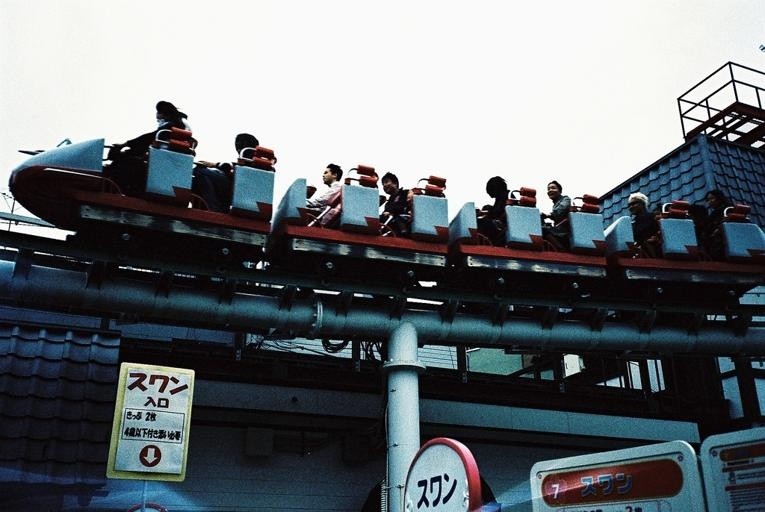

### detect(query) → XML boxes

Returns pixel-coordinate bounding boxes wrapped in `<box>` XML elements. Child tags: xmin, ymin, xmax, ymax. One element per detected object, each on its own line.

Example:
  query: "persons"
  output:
<box><xmin>652</xmin><ymin>208</ymin><xmax>662</xmax><ymax>227</ymax></box>
<box><xmin>694</xmin><ymin>189</ymin><xmax>737</xmax><ymax>262</ymax></box>
<box><xmin>192</xmin><ymin>134</ymin><xmax>259</xmax><ymax>213</ymax></box>
<box><xmin>380</xmin><ymin>172</ymin><xmax>416</xmax><ymax>237</ymax></box>
<box><xmin>541</xmin><ymin>181</ymin><xmax>573</xmax><ymax>252</ymax></box>
<box><xmin>628</xmin><ymin>192</ymin><xmax>662</xmax><ymax>258</ymax></box>
<box><xmin>307</xmin><ymin>163</ymin><xmax>343</xmax><ymax>226</ymax></box>
<box><xmin>478</xmin><ymin>175</ymin><xmax>509</xmax><ymax>247</ymax></box>
<box><xmin>104</xmin><ymin>102</ymin><xmax>190</xmax><ymax>195</ymax></box>
